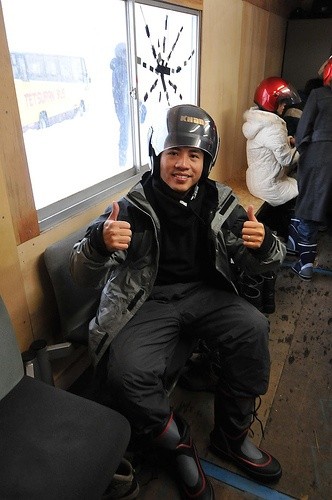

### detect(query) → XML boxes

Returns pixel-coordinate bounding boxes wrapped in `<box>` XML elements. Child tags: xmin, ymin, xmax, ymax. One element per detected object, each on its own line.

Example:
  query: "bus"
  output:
<box><xmin>9</xmin><ymin>51</ymin><xmax>91</xmax><ymax>133</ymax></box>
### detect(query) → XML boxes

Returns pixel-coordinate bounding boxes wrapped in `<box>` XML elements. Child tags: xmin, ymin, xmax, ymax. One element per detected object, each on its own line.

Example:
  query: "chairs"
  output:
<box><xmin>44</xmin><ymin>216</ymin><xmax>200</xmax><ymax>396</ymax></box>
<box><xmin>0</xmin><ymin>296</ymin><xmax>132</xmax><ymax>500</ymax></box>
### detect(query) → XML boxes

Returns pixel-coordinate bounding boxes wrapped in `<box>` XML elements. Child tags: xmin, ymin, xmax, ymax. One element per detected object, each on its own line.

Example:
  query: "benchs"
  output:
<box><xmin>223</xmin><ymin>167</ymin><xmax>266</xmax><ymax>217</ymax></box>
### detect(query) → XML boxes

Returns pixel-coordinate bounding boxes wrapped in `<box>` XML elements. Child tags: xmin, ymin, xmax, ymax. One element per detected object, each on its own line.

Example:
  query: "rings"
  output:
<box><xmin>246</xmin><ymin>235</ymin><xmax>251</xmax><ymax>242</ymax></box>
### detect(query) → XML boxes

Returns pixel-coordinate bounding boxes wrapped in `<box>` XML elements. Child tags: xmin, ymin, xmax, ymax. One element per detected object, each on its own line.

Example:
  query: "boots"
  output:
<box><xmin>291</xmin><ymin>242</ymin><xmax>317</xmax><ymax>280</ymax></box>
<box><xmin>261</xmin><ymin>270</ymin><xmax>276</xmax><ymax>314</ymax></box>
<box><xmin>241</xmin><ymin>277</ymin><xmax>261</xmax><ymax>310</ymax></box>
<box><xmin>284</xmin><ymin>219</ymin><xmax>300</xmax><ymax>254</ymax></box>
<box><xmin>144</xmin><ymin>413</ymin><xmax>215</xmax><ymax>499</ymax></box>
<box><xmin>208</xmin><ymin>389</ymin><xmax>282</xmax><ymax>483</ymax></box>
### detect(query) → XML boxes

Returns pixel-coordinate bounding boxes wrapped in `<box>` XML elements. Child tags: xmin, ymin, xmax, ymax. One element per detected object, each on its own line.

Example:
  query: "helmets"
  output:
<box><xmin>254</xmin><ymin>76</ymin><xmax>302</xmax><ymax>113</ymax></box>
<box><xmin>317</xmin><ymin>53</ymin><xmax>332</xmax><ymax>86</ymax></box>
<box><xmin>147</xmin><ymin>103</ymin><xmax>220</xmax><ymax>175</ymax></box>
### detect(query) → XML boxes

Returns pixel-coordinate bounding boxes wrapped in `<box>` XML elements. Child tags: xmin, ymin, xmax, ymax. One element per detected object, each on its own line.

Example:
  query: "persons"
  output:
<box><xmin>110</xmin><ymin>43</ymin><xmax>147</xmax><ymax>165</ymax></box>
<box><xmin>242</xmin><ymin>56</ymin><xmax>332</xmax><ymax>281</ymax></box>
<box><xmin>69</xmin><ymin>103</ymin><xmax>287</xmax><ymax>500</ymax></box>
